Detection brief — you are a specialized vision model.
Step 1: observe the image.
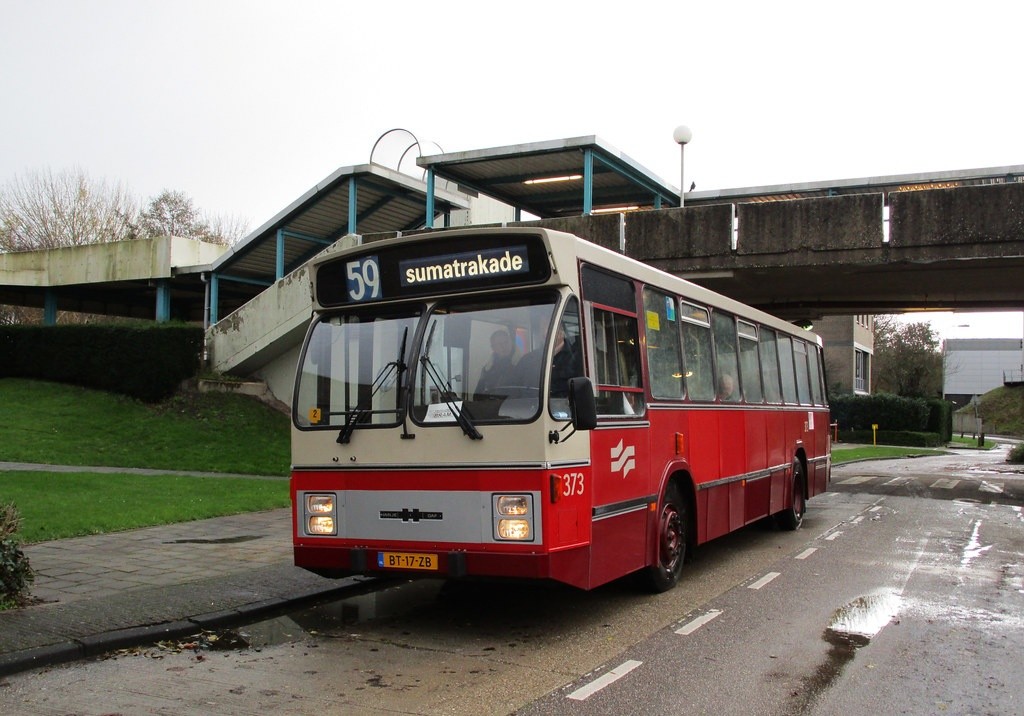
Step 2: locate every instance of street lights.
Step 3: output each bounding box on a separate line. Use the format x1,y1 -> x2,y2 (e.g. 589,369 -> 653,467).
673,125 -> 693,208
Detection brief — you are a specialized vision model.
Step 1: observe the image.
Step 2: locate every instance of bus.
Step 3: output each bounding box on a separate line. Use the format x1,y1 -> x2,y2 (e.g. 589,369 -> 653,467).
287,225 -> 833,596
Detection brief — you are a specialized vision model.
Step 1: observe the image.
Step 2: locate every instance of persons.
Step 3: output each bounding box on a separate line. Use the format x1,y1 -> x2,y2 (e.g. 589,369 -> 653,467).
473,317 -> 587,401
718,373 -> 738,400
